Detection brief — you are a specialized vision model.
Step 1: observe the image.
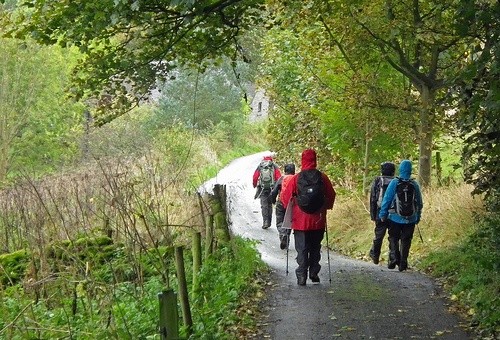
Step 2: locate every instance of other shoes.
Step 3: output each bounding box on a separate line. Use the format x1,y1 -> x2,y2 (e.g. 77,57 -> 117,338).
262,220 -> 268,229
309,273 -> 320,282
280,235 -> 287,249
399,264 -> 406,271
269,223 -> 271,226
369,249 -> 379,264
299,278 -> 305,286
388,258 -> 399,269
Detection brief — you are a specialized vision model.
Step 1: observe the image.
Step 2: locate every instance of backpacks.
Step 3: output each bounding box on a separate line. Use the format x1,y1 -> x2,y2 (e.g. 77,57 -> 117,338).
377,177 -> 395,212
259,162 -> 275,190
395,176 -> 416,217
293,170 -> 325,213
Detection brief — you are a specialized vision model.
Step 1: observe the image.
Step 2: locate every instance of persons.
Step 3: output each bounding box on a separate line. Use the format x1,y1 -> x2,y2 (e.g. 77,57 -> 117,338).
378,160 -> 422,272
280,149 -> 337,285
370,163 -> 400,266
270,163 -> 295,249
253,156 -> 281,229
